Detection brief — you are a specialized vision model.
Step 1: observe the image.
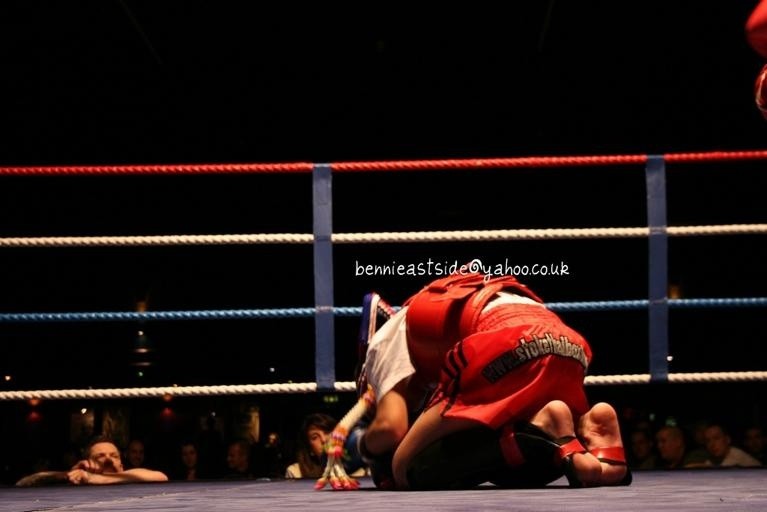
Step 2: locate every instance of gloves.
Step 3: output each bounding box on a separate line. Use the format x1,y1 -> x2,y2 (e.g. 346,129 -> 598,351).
346,427 -> 374,469
